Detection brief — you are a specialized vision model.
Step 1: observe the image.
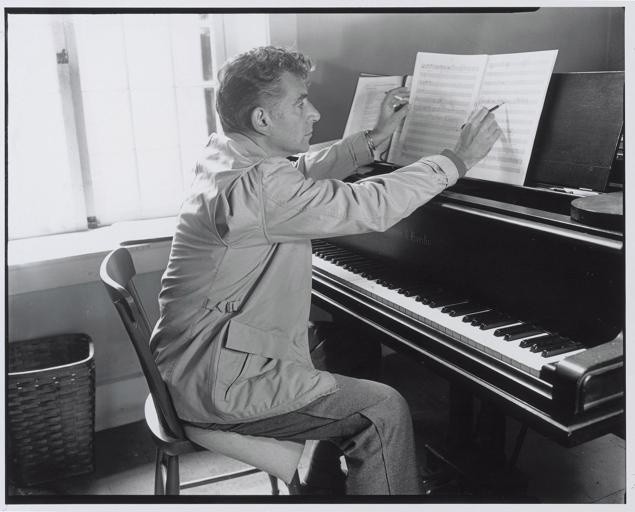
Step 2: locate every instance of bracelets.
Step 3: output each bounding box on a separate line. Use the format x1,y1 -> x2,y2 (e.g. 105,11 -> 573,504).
364,130 -> 376,150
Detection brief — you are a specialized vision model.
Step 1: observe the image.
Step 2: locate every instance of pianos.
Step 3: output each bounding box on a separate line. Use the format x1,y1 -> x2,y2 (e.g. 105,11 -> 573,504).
311,71 -> 626,503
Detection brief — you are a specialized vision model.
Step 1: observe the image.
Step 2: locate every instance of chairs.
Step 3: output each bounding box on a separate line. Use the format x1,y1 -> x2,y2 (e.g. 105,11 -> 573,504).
98,244 -> 318,498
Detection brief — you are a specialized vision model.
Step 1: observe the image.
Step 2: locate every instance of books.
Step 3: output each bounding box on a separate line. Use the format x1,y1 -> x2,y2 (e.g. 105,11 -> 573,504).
393,48 -> 560,186
342,72 -> 413,165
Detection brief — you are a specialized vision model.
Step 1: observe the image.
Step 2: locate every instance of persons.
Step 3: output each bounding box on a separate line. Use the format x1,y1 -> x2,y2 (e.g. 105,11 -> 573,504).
147,45 -> 503,498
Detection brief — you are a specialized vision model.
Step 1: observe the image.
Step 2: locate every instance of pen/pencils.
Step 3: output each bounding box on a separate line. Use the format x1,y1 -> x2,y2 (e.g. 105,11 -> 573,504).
460,102 -> 508,129
